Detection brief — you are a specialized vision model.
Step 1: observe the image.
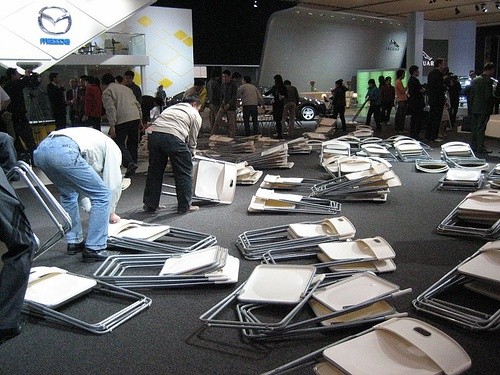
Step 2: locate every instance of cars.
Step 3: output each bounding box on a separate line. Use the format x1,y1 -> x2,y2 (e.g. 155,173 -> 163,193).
456,76 -> 471,107
163,91 -> 185,110
236,85 -> 326,121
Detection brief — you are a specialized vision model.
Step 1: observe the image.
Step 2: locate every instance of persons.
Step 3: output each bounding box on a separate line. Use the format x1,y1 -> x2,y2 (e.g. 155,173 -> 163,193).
394,70 -> 409,134
324,79 -> 348,134
143,94 -> 203,214
282,80 -> 299,138
307,81 -> 316,92
407,65 -> 427,141
365,76 -> 395,137
33,126 -> 123,263
207,70 -> 242,138
264,75 -> 288,138
464,62 -> 500,153
0,131 -> 36,344
141,95 -> 164,136
427,58 -> 462,142
171,85 -> 201,105
157,85 -> 167,112
1,68 -> 142,177
236,76 -> 263,136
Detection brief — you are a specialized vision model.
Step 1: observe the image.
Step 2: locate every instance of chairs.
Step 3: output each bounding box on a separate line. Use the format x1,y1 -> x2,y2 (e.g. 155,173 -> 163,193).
0,111 -> 500,375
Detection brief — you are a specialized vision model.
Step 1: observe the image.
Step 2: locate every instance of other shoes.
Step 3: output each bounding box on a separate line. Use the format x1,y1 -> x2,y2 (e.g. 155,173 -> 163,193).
143,203 -> 161,211
124,162 -> 139,178
178,205 -> 200,214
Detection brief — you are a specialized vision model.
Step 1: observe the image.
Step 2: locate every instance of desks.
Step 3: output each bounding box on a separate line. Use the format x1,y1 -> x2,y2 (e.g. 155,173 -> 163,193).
300,90 -> 332,102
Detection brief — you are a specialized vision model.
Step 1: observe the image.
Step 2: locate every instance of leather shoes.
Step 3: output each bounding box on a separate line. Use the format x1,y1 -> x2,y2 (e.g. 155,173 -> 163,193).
0,324 -> 23,345
82,249 -> 121,263
66,240 -> 86,255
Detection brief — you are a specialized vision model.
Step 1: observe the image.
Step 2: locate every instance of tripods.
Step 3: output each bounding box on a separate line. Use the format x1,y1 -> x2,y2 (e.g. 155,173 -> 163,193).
26,86 -> 50,145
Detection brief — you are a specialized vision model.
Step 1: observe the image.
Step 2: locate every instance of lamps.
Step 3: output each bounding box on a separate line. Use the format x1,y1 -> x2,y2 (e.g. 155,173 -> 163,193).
475,4 -> 480,10
454,7 -> 461,15
481,3 -> 489,12
495,1 -> 500,10
